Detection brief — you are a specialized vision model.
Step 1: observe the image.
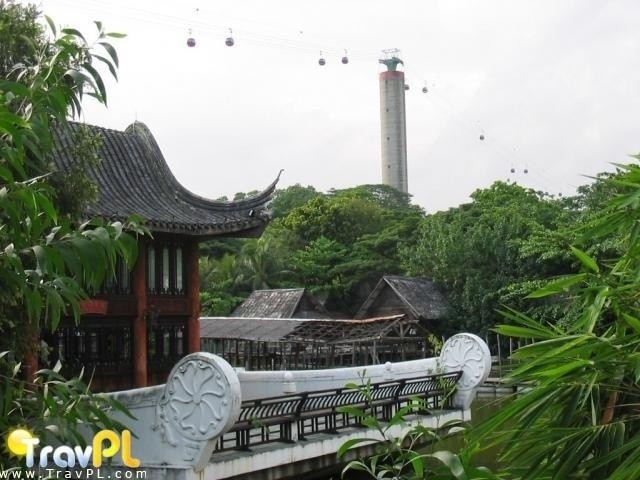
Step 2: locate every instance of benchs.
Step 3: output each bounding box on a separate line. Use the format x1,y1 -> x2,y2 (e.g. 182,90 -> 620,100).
213,370 -> 466,456
